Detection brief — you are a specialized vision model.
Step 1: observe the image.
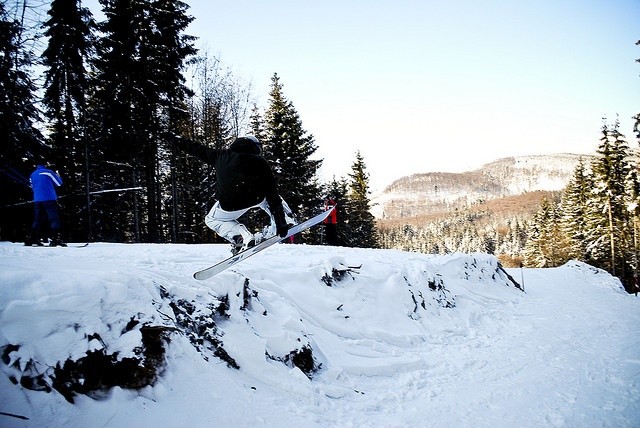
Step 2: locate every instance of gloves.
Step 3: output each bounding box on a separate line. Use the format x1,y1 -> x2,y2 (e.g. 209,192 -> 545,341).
159,130 -> 183,148
276,220 -> 291,237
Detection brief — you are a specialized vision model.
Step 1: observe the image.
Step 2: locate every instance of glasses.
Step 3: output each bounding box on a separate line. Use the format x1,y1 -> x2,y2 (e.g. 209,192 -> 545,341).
254,139 -> 264,144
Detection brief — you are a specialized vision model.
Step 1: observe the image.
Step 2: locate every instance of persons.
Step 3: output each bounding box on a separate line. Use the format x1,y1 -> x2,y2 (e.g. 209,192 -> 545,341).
25,158 -> 64,246
159,131 -> 298,256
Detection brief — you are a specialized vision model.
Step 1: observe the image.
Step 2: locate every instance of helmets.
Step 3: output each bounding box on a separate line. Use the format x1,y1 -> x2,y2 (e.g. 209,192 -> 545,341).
239,135 -> 260,142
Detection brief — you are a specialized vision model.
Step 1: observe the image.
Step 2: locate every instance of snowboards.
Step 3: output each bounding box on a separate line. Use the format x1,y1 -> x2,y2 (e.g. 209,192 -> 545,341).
23,242 -> 89,247
194,202 -> 337,281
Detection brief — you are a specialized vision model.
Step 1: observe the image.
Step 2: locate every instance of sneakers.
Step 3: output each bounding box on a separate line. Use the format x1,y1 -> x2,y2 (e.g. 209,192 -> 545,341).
230,234 -> 255,256
28,238 -> 44,245
49,239 -> 66,246
261,213 -> 295,241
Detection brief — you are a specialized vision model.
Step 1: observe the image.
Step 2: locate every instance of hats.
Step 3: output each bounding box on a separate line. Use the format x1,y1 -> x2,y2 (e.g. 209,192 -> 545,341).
35,158 -> 47,166
